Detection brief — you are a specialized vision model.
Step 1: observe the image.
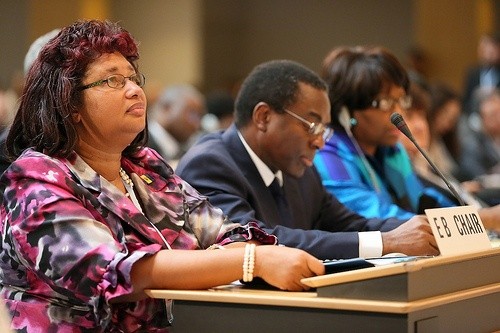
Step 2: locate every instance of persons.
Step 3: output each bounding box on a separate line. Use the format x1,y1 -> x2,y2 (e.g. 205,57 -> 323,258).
177,59 -> 440,262
0,18 -> 325,333
313,46 -> 500,235
0,29 -> 500,210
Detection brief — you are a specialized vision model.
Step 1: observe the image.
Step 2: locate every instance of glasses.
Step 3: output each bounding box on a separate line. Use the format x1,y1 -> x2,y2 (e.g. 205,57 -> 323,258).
76,73 -> 146,92
367,95 -> 412,112
282,108 -> 334,143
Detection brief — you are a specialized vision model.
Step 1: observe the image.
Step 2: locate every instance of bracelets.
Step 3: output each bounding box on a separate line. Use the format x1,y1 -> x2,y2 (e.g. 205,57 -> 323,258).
205,243 -> 224,250
243,243 -> 256,282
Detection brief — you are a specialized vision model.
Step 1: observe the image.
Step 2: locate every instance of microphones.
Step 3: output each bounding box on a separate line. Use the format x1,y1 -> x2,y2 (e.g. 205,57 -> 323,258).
389,112 -> 465,206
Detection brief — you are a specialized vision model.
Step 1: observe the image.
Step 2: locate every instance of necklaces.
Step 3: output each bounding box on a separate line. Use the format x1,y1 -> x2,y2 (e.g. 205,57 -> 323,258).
119,167 -> 134,199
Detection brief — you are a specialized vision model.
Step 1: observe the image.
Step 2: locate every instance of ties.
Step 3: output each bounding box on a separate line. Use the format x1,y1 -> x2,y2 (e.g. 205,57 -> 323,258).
268,176 -> 290,226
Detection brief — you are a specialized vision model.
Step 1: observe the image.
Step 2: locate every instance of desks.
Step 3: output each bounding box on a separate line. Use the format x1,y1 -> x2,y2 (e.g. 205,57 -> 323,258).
151,248 -> 500,333
